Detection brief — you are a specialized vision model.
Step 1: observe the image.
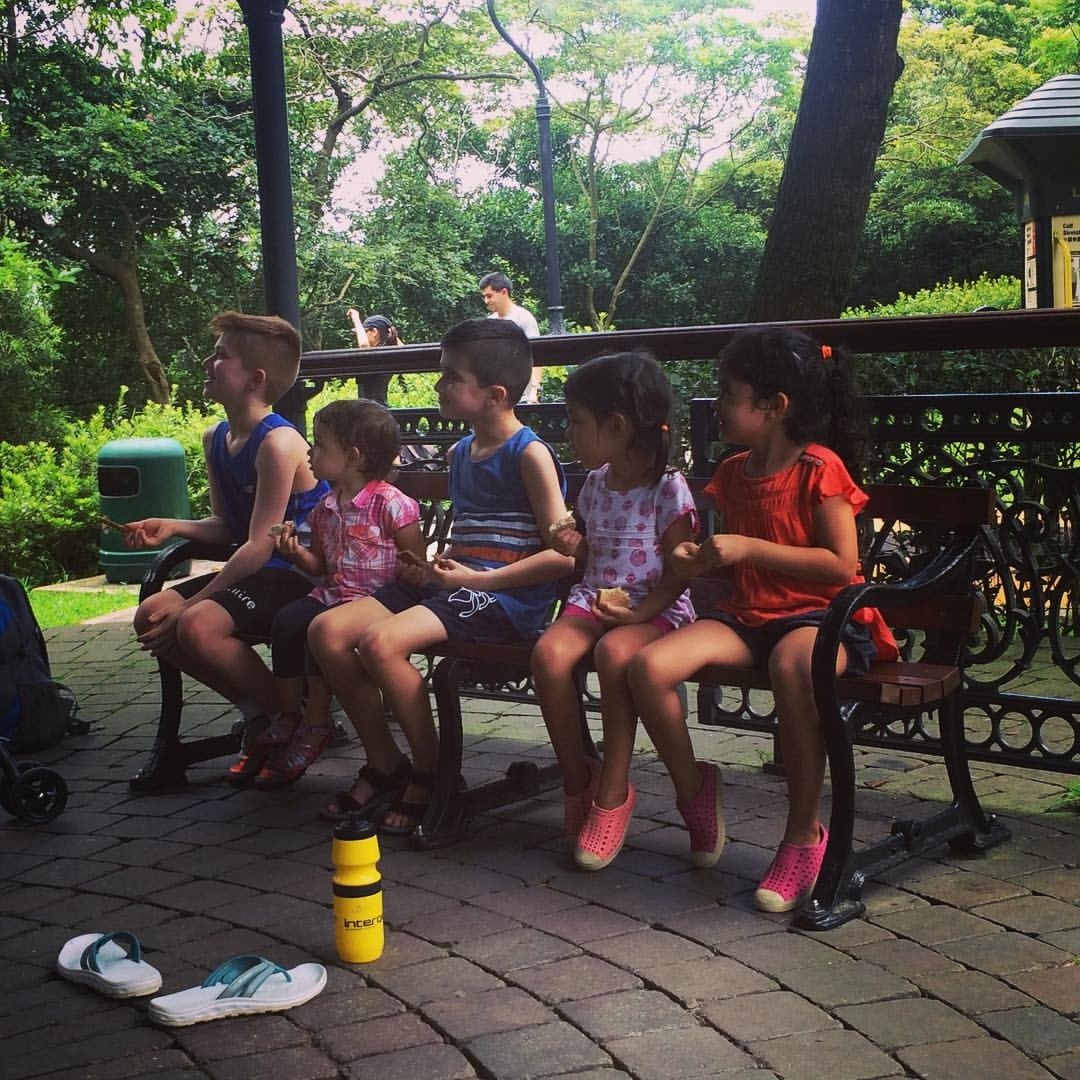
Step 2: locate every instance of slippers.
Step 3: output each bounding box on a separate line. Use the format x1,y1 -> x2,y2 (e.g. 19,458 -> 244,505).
57,931 -> 162,1001
149,954 -> 328,1028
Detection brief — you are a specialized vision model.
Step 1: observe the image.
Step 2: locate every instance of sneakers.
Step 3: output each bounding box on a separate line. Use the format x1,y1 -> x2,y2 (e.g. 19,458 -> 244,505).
228,714 -> 272,781
253,712 -> 304,793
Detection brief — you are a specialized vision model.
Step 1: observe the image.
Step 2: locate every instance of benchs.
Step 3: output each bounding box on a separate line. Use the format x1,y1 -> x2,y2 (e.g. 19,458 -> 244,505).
129,472 -> 996,933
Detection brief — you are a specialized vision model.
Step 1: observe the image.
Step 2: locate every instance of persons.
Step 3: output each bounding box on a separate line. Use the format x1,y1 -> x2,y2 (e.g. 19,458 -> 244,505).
479,271 -> 543,405
346,307 -> 407,408
627,327 -> 899,913
531,351 -> 695,873
308,319 -> 577,833
122,310 -> 331,789
256,400 -> 427,772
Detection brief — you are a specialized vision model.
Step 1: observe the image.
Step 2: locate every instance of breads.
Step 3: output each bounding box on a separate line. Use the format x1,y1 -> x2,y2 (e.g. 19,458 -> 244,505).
268,520 -> 297,539
548,516 -> 577,535
597,586 -> 632,610
396,549 -> 421,565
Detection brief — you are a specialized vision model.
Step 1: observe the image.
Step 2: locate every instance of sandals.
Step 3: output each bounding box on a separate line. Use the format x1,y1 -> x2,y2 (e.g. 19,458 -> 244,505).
318,753 -> 412,818
379,771 -> 435,833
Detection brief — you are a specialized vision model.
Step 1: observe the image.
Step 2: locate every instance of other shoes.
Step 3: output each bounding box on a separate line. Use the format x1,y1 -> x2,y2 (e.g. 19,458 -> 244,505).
267,707 -> 308,746
564,760 -> 603,842
572,783 -> 635,870
283,718 -> 336,770
753,823 -> 829,912
676,762 -> 725,868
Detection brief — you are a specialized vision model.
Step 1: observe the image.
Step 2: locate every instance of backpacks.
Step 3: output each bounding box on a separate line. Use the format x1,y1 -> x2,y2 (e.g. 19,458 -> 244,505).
0,574 -> 89,754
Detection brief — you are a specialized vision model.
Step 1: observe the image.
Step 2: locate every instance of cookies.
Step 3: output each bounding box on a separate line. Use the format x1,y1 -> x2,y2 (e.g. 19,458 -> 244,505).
97,516 -> 125,529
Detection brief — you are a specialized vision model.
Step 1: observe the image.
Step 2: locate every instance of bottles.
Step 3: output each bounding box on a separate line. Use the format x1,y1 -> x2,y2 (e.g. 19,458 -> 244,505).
330,808 -> 385,965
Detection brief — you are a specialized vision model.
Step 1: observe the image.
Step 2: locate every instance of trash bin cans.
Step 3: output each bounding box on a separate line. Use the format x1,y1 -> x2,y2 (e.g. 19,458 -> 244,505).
94,437 -> 193,581
